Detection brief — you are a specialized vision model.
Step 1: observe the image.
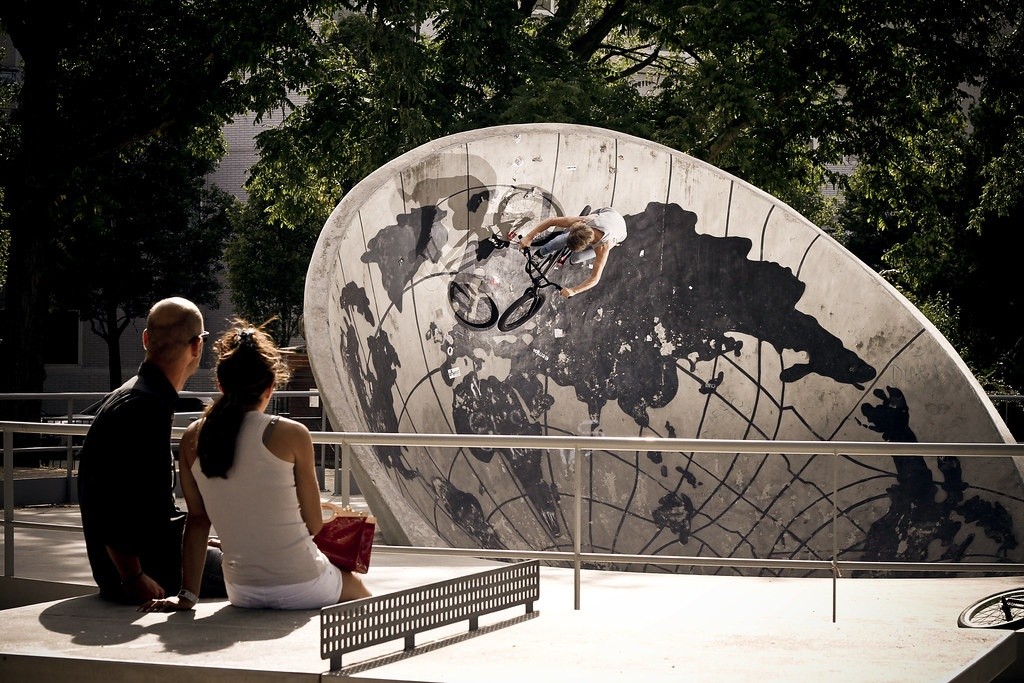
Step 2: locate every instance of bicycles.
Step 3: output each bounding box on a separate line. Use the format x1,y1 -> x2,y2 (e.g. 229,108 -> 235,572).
498,205 -> 591,332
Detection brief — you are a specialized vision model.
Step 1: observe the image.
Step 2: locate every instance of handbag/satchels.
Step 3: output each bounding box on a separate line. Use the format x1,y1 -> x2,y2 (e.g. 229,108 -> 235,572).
308,502 -> 377,574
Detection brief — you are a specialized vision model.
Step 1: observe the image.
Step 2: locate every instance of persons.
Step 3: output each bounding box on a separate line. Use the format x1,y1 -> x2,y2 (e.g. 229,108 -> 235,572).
516,206 -> 629,298
77,296 -> 373,613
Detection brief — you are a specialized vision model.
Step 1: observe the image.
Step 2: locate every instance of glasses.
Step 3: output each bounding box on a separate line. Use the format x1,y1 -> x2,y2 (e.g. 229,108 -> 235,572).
188,331 -> 210,345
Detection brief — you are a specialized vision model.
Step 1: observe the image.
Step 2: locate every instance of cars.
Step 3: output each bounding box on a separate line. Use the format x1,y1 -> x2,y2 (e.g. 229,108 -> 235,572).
48,390 -> 215,450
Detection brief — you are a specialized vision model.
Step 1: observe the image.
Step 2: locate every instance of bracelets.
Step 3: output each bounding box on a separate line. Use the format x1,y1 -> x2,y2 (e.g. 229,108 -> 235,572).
120,572 -> 143,583
177,588 -> 199,604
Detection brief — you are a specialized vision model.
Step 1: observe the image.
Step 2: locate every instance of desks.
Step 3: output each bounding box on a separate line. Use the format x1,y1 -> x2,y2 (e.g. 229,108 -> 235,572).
0,551 -> 1024,683
